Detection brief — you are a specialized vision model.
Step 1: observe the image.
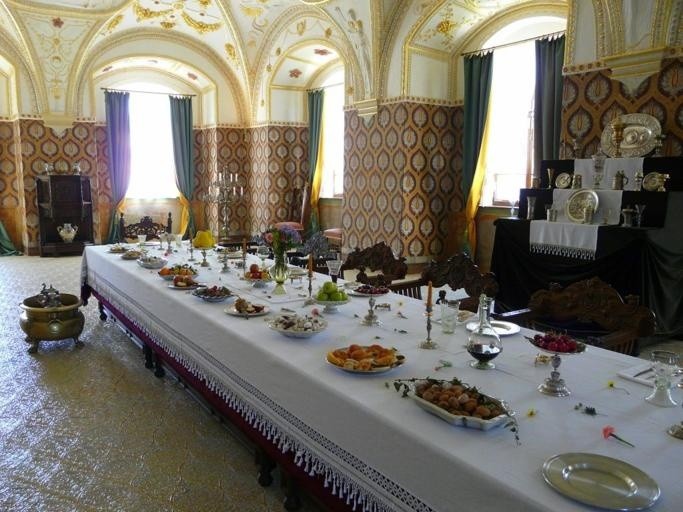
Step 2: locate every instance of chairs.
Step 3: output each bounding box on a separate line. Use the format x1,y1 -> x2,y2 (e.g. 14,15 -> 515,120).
119,211 -> 172,242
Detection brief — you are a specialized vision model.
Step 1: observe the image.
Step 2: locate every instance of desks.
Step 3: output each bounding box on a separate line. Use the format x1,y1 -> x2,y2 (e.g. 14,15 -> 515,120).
78,238 -> 683,512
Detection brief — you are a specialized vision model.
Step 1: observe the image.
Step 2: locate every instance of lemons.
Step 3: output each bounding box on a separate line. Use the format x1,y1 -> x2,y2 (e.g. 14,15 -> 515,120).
193,230 -> 214,247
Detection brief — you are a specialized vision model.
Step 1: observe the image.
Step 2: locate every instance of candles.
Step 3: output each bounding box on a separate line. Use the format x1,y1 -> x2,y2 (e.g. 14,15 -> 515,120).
208,172 -> 244,197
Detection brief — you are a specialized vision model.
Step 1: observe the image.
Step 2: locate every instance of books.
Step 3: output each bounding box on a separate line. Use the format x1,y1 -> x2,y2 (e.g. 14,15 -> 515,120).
616,361 -> 682,389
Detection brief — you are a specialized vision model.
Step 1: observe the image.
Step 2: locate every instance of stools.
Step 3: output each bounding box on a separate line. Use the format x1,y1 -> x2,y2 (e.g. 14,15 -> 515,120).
274,221 -> 305,240
324,227 -> 342,252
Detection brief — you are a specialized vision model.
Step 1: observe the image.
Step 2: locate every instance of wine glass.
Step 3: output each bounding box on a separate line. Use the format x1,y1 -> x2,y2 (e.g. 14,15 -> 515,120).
525,169 -> 670,229
590,154 -> 606,189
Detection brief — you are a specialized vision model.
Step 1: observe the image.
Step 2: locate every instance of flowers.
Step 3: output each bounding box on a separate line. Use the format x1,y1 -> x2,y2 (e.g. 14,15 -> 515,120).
259,222 -> 303,277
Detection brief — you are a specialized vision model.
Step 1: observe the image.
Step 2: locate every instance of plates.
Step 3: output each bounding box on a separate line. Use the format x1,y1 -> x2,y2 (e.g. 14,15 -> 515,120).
410,379 -> 514,433
465,320 -> 523,336
312,294 -> 352,314
541,452 -> 661,510
326,345 -> 407,376
600,113 -> 662,158
530,340 -> 589,396
350,286 -> 390,327
269,314 -> 328,340
223,303 -> 271,317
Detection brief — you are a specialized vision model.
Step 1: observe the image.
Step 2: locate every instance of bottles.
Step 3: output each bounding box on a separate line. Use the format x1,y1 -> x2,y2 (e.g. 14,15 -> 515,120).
467,296 -> 505,370
72,162 -> 82,176
44,164 -> 56,176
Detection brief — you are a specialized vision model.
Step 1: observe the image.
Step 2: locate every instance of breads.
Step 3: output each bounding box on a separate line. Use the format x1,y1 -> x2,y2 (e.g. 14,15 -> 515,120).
160,268 -> 169,274
328,344 -> 394,371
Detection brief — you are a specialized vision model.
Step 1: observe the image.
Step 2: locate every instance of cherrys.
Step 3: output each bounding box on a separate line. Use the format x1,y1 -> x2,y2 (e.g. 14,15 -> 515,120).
356,281 -> 387,294
534,329 -> 581,352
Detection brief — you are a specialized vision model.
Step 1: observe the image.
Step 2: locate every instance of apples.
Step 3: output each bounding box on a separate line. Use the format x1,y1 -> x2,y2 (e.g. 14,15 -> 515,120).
316,283 -> 348,302
245,264 -> 269,279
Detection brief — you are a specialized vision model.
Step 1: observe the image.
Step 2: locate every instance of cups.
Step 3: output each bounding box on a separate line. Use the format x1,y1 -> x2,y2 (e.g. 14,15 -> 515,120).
645,349 -> 681,408
439,300 -> 462,334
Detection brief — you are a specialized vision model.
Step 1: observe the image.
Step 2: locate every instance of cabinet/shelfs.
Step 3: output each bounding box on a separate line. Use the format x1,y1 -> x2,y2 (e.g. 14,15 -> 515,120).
35,173 -> 94,257
491,156 -> 683,357
